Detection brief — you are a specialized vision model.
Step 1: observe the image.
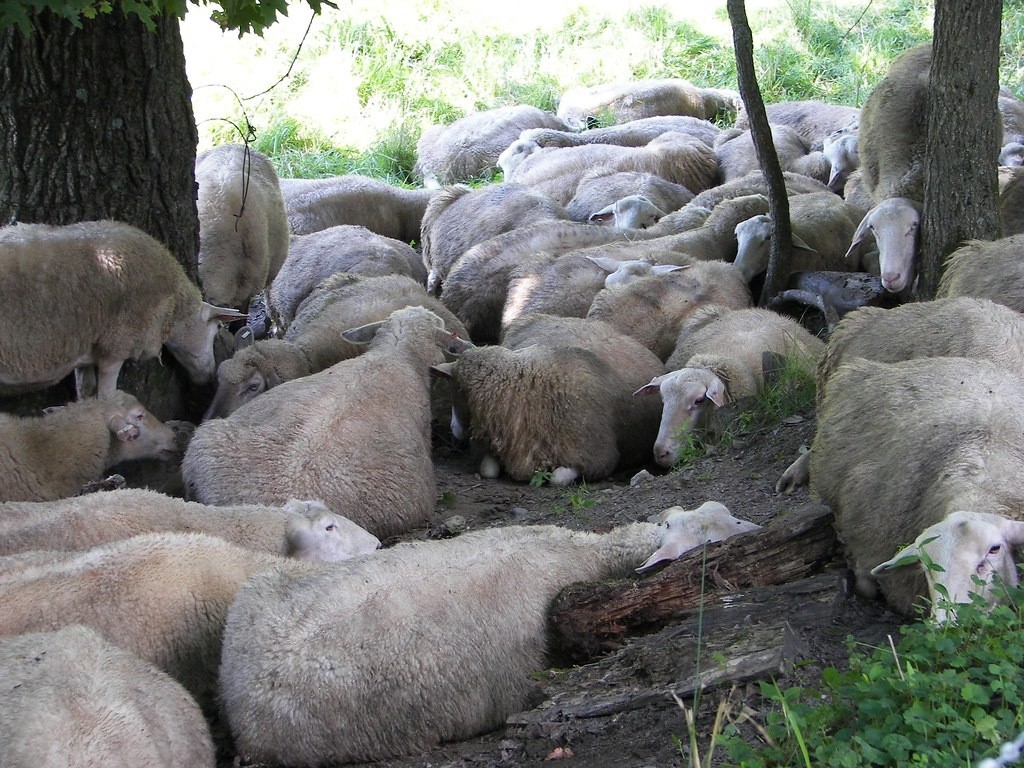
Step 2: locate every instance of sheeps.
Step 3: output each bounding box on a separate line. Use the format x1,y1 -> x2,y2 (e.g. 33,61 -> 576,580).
0,42 -> 1024,768
